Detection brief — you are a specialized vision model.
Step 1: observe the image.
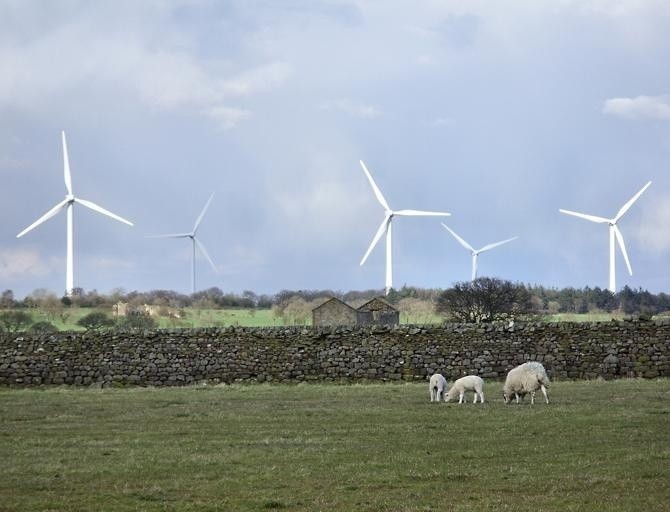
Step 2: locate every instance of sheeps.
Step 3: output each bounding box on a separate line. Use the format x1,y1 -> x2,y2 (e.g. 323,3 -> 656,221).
444,375 -> 485,404
429,373 -> 449,404
502,362 -> 553,407
